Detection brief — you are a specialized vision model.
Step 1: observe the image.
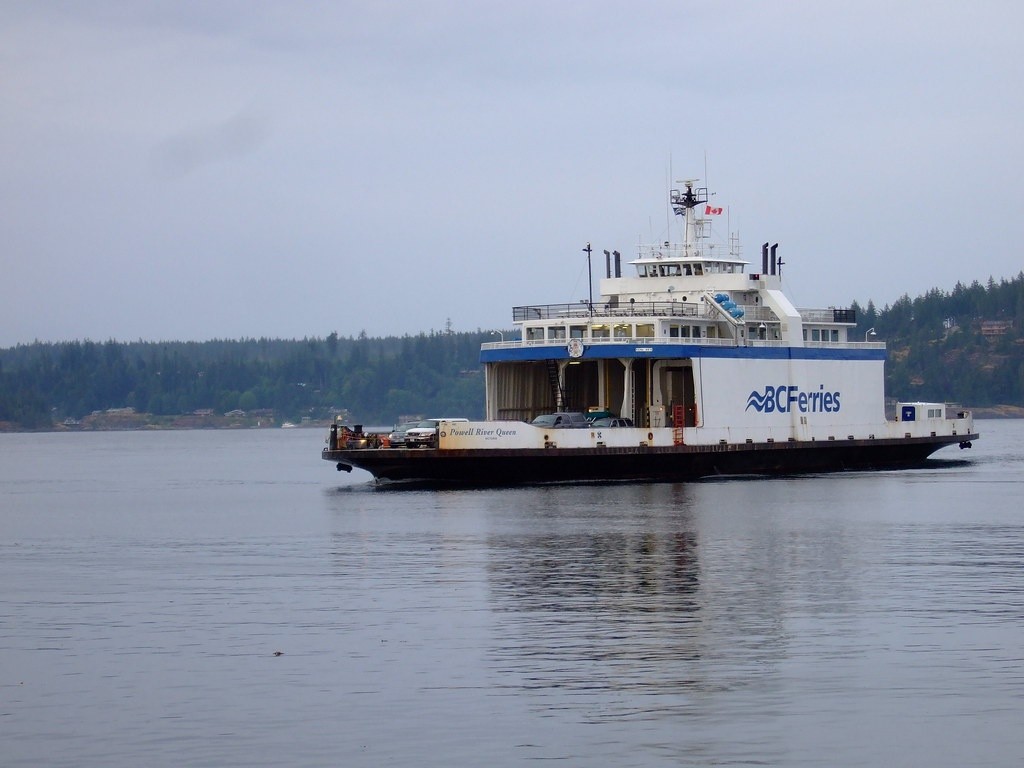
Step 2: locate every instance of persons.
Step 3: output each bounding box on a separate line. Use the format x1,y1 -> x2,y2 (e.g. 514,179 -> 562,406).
675,265 -> 681,273
660,266 -> 664,275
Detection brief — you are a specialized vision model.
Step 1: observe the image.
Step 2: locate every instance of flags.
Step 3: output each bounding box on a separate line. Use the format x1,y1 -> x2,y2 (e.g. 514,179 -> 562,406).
705,205 -> 722,215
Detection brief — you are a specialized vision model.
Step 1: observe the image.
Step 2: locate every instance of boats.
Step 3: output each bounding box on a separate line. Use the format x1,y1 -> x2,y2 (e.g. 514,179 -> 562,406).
322,149 -> 980,484
